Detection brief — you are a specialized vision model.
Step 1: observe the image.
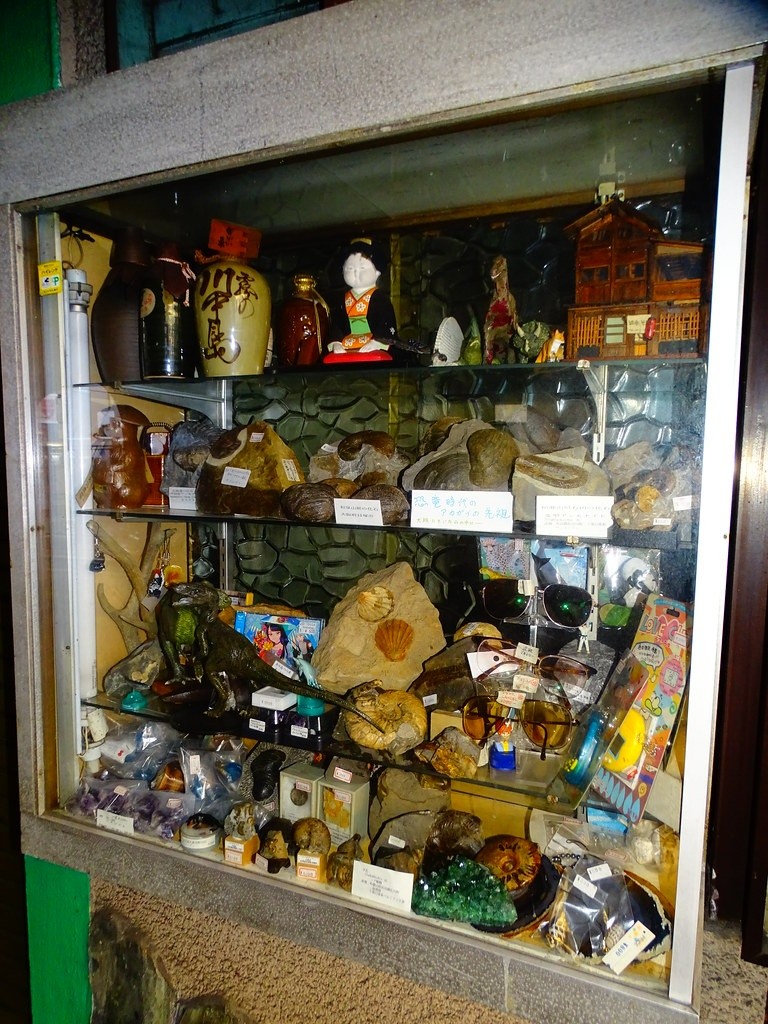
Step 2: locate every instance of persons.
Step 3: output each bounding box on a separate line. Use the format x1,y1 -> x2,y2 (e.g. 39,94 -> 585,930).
322,238 -> 396,363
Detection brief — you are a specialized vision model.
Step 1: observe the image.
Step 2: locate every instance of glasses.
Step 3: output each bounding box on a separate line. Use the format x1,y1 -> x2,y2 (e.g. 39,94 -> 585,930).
481,578 -> 592,628
476,637 -> 598,700
462,695 -> 583,760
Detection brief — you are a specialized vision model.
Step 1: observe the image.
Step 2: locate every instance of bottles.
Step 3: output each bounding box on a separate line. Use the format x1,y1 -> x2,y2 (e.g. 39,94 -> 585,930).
90,225 -> 330,384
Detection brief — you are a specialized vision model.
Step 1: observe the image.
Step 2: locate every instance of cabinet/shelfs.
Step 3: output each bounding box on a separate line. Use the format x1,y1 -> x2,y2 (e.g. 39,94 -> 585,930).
50,61 -> 728,989
